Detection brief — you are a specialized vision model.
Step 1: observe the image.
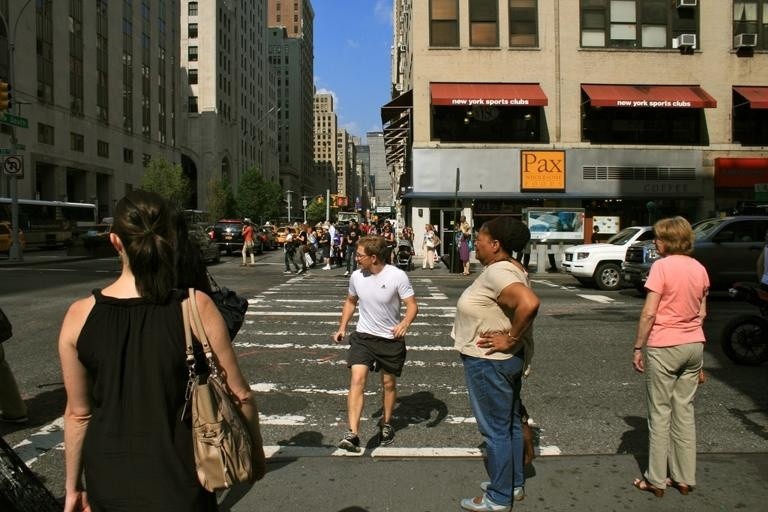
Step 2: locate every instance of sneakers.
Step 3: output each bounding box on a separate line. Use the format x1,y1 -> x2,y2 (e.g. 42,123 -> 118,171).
461,493 -> 512,511
378,423 -> 393,446
480,482 -> 524,500
283,265 -> 350,276
338,432 -> 362,452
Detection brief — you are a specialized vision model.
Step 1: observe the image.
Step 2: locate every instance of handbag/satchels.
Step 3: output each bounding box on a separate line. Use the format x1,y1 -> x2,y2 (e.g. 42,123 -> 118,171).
246,240 -> 254,249
207,286 -> 248,340
188,355 -> 254,493
522,421 -> 535,466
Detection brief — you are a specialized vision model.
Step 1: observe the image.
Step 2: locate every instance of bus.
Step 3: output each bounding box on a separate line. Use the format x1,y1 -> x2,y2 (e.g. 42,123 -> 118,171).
0,198 -> 98,252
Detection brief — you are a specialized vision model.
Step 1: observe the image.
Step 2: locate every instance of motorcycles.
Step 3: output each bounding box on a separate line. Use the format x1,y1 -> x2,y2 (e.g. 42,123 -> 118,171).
720,280 -> 767,365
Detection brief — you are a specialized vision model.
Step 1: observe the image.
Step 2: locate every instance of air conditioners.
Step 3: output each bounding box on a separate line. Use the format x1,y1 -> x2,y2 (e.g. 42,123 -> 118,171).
676,0 -> 697,9
678,34 -> 695,49
395,0 -> 409,92
733,33 -> 758,49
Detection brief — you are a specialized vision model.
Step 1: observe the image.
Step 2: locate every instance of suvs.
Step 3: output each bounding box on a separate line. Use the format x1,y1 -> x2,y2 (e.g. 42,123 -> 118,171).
621,216 -> 767,291
561,224 -> 656,290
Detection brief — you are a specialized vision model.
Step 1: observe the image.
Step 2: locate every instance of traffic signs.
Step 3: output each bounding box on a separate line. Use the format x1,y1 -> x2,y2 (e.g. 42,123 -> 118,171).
0,114 -> 27,129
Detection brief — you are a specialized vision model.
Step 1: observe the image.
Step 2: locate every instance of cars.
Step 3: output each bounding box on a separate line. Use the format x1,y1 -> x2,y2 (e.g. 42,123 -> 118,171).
0,221 -> 27,257
182,216 -> 309,265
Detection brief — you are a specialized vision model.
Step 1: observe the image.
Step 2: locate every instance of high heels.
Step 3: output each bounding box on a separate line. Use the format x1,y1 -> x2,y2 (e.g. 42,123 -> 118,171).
666,477 -> 689,495
633,477 -> 664,497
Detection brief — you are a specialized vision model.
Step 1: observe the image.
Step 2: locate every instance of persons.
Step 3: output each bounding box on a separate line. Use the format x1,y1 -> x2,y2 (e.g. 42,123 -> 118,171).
238,215 -> 533,276
590,225 -> 600,243
754,241 -> 768,293
554,212 -> 579,230
163,212 -> 212,428
333,235 -> 418,453
58,188 -> 265,511
0,308 -> 29,423
631,216 -> 711,497
450,216 -> 541,512
519,402 -> 537,470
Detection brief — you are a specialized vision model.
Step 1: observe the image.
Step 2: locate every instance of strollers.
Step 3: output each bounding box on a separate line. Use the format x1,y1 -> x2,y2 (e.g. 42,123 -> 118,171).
393,238 -> 414,270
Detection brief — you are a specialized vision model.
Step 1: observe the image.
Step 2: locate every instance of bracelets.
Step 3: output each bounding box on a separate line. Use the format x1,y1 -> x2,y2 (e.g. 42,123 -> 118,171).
507,330 -> 521,341
634,346 -> 642,352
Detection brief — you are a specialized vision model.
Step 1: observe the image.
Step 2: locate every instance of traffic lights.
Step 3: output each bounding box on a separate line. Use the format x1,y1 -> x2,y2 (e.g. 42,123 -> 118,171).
335,196 -> 348,207
0,83 -> 12,111
